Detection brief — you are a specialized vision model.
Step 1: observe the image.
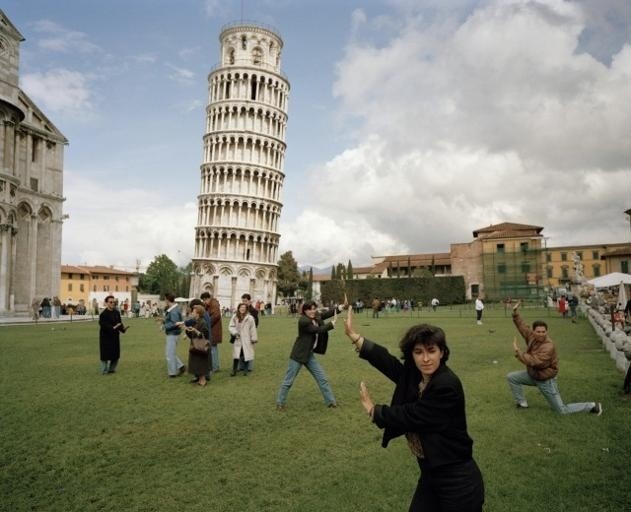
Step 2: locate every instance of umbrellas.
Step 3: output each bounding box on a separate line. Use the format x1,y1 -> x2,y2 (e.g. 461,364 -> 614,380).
586,272 -> 631,288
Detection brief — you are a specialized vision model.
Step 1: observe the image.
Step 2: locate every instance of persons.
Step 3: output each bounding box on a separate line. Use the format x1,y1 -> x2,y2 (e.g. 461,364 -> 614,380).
345,304 -> 485,511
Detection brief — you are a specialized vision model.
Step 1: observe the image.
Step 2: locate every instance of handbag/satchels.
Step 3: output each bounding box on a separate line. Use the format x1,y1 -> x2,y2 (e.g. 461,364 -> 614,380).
193,333 -> 208,351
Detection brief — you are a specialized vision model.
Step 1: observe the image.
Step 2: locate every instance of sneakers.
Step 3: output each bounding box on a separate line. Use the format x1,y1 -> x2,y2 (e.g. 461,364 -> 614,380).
591,402 -> 601,416
